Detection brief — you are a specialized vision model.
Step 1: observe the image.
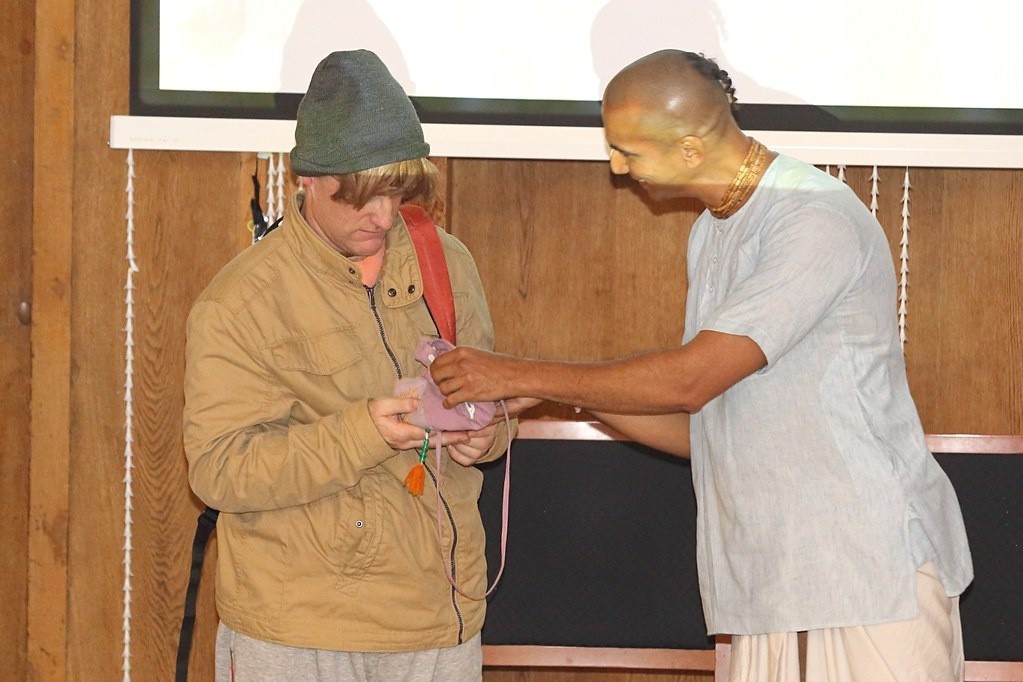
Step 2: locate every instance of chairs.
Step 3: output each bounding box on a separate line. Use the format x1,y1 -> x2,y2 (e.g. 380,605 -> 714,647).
472,415 -> 1023,682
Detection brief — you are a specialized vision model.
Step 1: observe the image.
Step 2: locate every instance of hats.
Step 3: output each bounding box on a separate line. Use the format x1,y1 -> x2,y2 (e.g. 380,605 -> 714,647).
289,48 -> 431,178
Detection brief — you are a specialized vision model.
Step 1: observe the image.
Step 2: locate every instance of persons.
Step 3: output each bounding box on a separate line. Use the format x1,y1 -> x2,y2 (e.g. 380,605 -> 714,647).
177,50 -> 518,682
431,48 -> 978,682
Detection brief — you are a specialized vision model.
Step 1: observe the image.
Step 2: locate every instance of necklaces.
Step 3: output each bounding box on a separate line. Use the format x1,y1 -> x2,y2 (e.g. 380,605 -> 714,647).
703,134 -> 769,219
